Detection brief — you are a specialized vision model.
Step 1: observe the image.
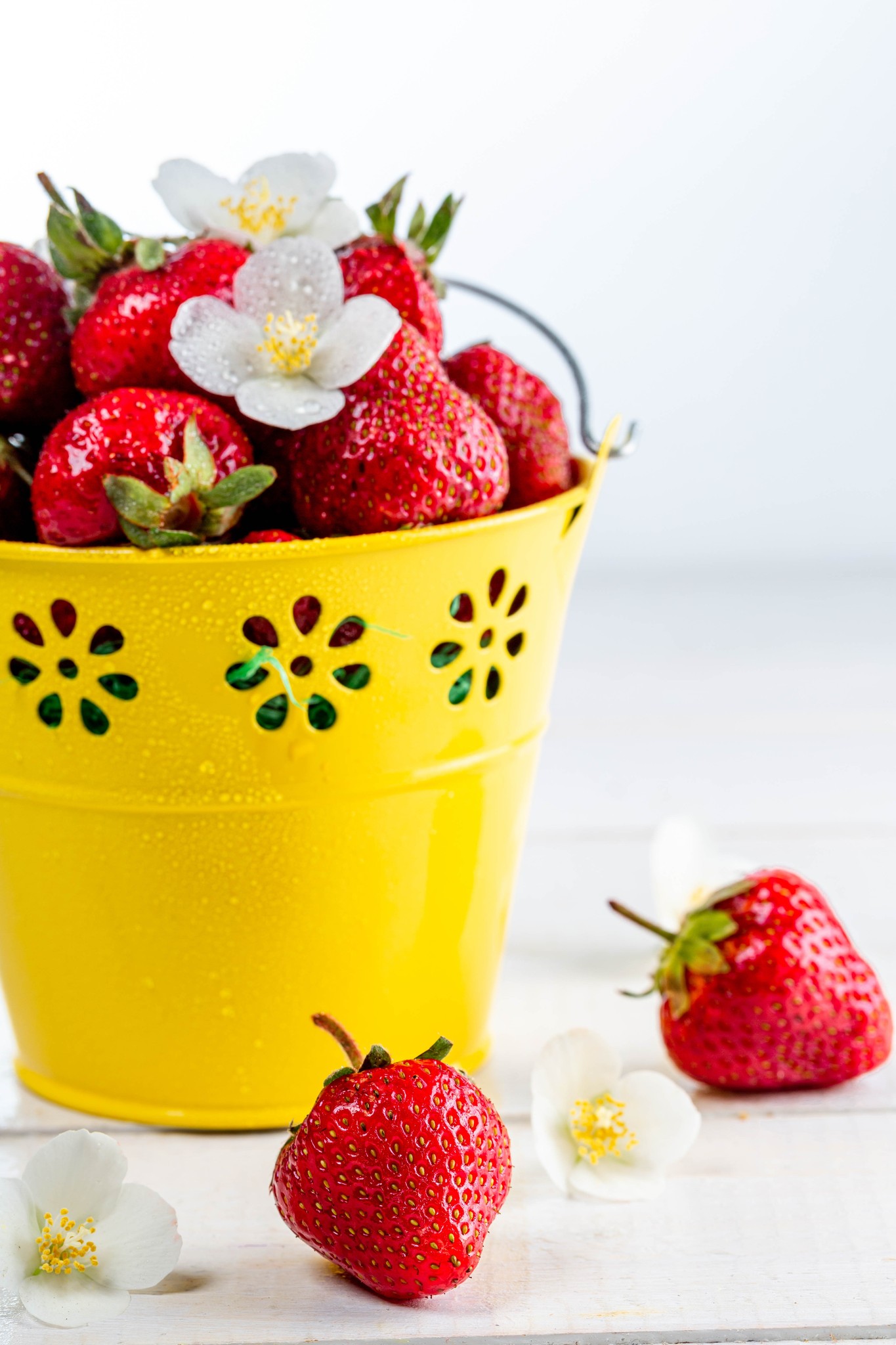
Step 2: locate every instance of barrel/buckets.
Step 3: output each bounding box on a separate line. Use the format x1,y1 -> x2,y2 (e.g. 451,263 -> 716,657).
1,270 -> 638,1131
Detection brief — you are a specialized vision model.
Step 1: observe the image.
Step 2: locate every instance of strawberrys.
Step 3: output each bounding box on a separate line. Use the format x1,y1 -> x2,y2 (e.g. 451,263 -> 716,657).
605,870 -> 896,1094
0,173 -> 577,672
266,1016 -> 512,1299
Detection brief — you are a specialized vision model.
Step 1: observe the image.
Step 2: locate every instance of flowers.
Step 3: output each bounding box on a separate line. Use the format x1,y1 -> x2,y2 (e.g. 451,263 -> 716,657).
530,1029 -> 702,1197
154,147 -> 399,426
0,1129 -> 180,1327
